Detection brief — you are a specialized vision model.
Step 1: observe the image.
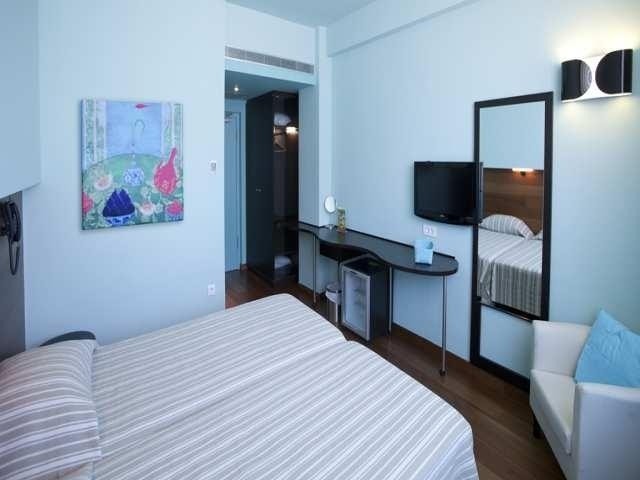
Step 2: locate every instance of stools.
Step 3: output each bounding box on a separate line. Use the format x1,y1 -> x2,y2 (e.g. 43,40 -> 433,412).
528,319 -> 638,480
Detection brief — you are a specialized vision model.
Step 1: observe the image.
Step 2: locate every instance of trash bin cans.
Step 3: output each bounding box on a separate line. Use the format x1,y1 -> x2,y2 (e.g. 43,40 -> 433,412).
325,281 -> 342,324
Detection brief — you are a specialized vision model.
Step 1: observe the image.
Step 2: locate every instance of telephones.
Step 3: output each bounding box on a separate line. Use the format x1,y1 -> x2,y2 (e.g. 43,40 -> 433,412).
0,201 -> 21,240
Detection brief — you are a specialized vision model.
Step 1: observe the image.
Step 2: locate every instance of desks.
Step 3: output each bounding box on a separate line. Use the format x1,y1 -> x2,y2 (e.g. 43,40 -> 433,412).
312,224 -> 458,376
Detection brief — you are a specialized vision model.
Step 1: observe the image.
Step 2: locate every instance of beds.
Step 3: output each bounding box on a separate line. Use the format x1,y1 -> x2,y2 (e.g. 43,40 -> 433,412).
0,293 -> 472,480
478,167 -> 544,315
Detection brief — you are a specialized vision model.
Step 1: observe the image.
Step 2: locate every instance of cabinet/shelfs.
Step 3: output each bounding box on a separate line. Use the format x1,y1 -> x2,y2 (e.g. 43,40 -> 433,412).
244,91 -> 299,291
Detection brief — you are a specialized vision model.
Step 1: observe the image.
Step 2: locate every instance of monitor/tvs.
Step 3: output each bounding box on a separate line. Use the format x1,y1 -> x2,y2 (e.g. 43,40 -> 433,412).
413,161 -> 484,225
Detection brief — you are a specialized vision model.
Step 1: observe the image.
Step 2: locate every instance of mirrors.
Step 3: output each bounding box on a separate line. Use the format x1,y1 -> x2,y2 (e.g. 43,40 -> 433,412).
468,91 -> 554,391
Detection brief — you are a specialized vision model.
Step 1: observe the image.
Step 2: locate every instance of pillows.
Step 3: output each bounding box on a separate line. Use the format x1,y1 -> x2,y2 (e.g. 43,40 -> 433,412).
479,213 -> 533,241
0,335 -> 103,480
574,306 -> 638,389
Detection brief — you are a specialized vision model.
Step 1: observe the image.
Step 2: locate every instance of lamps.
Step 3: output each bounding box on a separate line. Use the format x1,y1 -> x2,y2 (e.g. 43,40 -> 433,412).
559,46 -> 634,105
